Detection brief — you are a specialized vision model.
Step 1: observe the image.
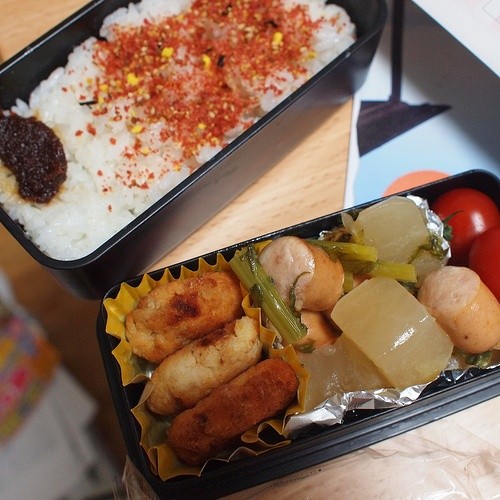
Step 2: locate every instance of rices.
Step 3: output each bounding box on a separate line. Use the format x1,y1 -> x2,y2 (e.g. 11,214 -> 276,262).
0,1 -> 353,261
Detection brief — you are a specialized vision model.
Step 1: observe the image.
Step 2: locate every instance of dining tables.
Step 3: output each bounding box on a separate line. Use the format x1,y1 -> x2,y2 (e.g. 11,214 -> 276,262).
1,1 -> 500,500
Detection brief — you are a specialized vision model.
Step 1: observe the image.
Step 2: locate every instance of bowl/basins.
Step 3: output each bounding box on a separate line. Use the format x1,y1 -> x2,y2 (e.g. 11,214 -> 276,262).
0,0 -> 401,303
95,166 -> 499,499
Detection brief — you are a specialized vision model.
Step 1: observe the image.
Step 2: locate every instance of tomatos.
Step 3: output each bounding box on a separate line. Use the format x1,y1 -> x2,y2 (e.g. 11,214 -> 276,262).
436,187 -> 500,301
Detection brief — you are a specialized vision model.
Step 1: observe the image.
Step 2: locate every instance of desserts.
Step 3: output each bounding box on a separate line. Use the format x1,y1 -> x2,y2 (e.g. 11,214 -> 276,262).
126,270 -> 299,465
